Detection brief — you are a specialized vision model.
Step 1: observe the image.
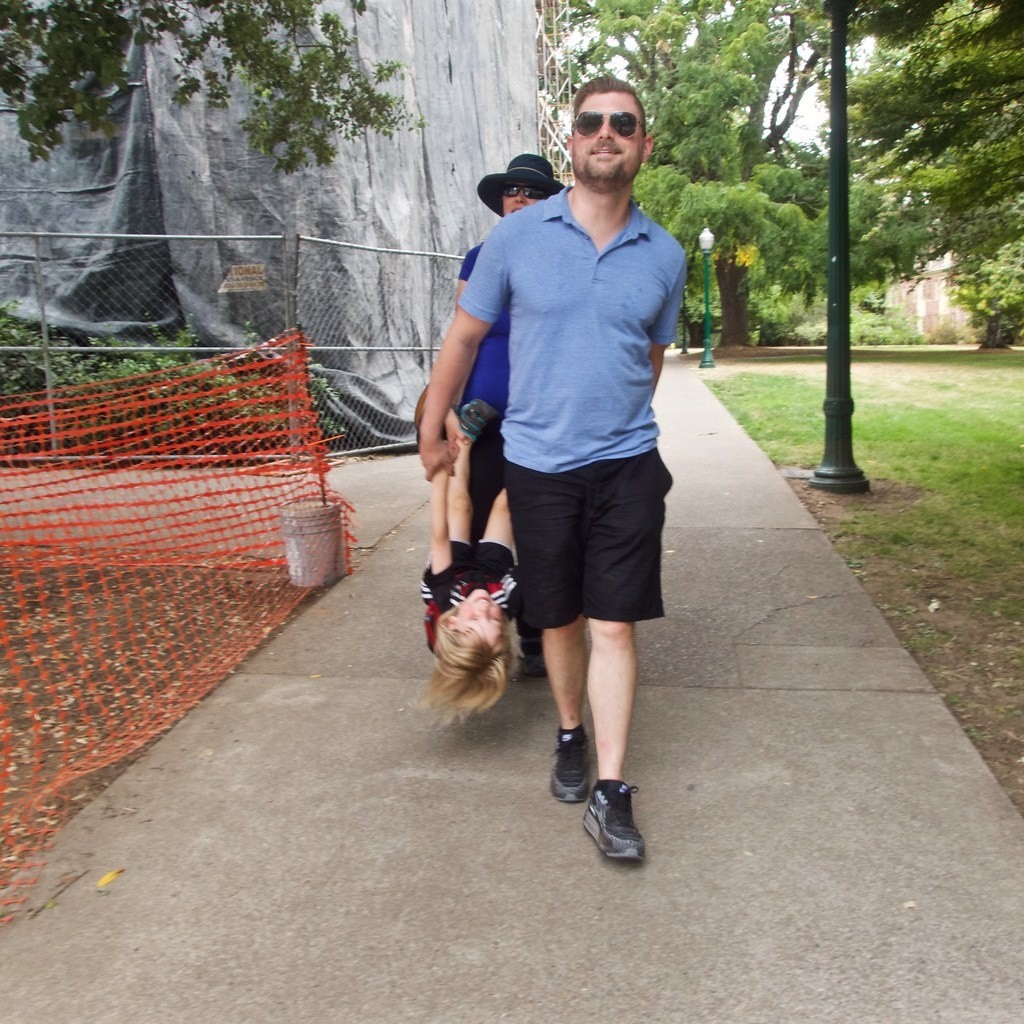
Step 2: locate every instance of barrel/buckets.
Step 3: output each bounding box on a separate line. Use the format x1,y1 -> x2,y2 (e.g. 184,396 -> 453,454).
279,496 -> 347,588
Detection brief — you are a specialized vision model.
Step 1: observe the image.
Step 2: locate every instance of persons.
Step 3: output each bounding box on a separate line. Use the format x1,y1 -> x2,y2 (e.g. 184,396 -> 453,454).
413,153 -> 568,726
420,76 -> 687,861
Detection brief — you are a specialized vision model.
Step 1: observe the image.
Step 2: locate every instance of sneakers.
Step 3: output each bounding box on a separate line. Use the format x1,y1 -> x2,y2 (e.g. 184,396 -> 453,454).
581,782 -> 645,859
549,729 -> 591,803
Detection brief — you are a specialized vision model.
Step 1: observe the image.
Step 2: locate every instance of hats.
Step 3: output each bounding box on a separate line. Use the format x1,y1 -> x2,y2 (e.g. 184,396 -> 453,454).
477,153 -> 566,220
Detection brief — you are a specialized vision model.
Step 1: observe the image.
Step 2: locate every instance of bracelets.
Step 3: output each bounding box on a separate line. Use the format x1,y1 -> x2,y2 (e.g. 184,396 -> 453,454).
451,403 -> 461,416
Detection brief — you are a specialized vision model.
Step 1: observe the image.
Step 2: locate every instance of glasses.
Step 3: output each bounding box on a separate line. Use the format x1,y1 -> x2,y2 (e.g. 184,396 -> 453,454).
499,184 -> 548,199
571,110 -> 646,140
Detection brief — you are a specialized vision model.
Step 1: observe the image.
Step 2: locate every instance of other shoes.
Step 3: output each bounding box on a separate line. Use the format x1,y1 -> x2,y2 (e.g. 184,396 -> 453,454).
519,635 -> 548,678
460,397 -> 500,441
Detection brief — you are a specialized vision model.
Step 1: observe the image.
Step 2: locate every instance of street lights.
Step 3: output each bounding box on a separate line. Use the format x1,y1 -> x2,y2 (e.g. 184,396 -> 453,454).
701,227 -> 716,368
